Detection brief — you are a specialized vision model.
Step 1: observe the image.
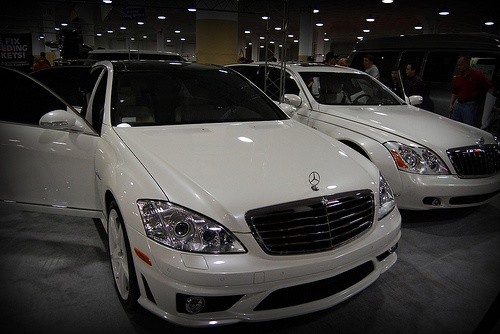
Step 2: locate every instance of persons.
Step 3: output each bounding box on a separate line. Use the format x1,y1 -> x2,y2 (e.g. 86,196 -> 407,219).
307,56 -> 313,62
239,57 -> 251,64
273,58 -> 277,62
307,51 -> 379,104
448,54 -> 494,126
35,52 -> 51,71
390,62 -> 434,112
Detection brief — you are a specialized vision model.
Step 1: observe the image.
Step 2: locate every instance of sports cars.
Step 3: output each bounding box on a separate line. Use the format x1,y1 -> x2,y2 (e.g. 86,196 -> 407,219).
1,43 -> 402,334
221,59 -> 500,215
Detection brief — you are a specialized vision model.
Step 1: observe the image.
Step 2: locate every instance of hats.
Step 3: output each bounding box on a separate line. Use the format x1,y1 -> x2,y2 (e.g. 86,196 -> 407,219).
326,51 -> 339,58
